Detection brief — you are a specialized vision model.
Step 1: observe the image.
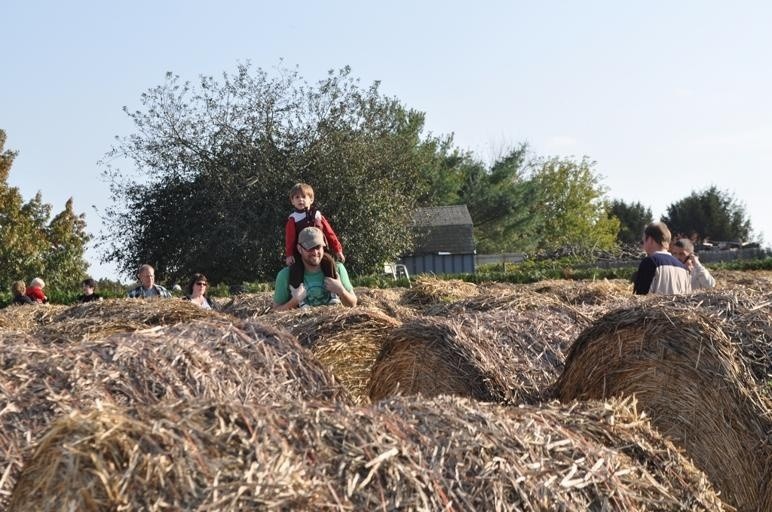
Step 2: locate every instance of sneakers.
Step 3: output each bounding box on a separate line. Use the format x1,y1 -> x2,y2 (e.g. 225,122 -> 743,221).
330,292 -> 341,303
299,301 -> 309,308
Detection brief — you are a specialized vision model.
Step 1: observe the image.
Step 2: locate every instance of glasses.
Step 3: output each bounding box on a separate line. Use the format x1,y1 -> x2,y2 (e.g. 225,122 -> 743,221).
194,281 -> 209,287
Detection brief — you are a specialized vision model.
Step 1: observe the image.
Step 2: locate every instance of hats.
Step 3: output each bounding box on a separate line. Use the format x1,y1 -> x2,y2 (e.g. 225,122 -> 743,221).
297,226 -> 327,250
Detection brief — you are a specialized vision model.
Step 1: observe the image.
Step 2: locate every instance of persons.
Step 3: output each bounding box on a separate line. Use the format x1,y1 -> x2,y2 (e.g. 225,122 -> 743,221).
76,277 -> 100,302
11,280 -> 34,305
179,271 -> 213,311
26,277 -> 49,304
634,221 -> 693,295
284,182 -> 345,308
272,225 -> 358,311
671,238 -> 717,291
123,263 -> 172,298
